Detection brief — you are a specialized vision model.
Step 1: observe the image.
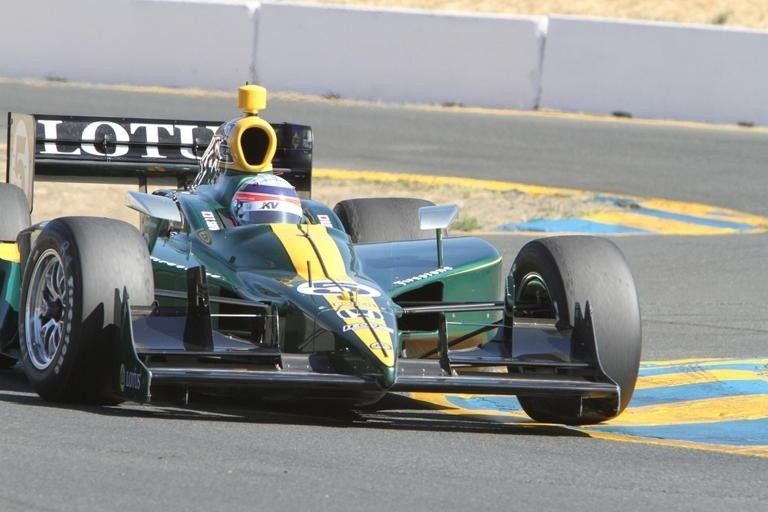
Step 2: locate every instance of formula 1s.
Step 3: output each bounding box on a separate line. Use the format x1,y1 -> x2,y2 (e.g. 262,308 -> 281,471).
2,83 -> 641,427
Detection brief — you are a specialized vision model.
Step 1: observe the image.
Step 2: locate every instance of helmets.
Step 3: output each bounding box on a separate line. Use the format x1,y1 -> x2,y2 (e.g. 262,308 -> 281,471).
230,174 -> 303,225
